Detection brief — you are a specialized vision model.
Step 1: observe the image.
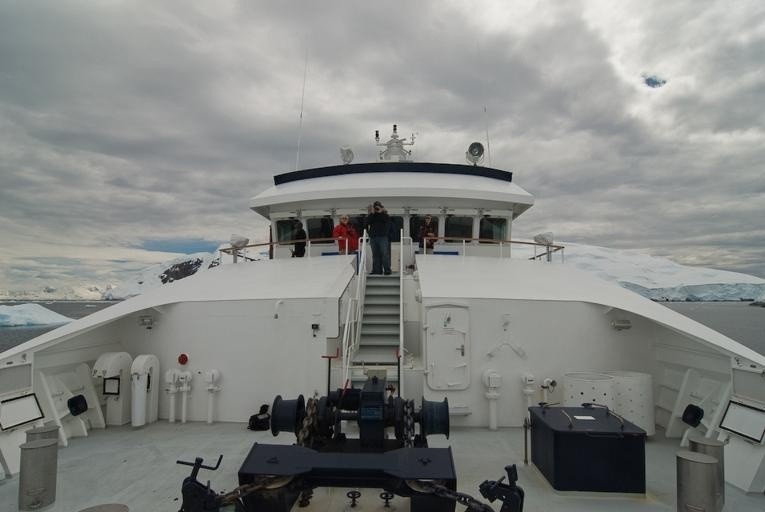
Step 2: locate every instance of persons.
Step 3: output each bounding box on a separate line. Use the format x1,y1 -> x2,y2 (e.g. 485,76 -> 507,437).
417,214 -> 438,254
365,201 -> 392,275
332,214 -> 358,255
292,222 -> 306,257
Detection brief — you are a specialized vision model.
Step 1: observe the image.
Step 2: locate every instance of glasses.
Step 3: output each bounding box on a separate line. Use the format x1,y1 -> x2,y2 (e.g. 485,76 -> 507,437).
425,217 -> 430,219
342,218 -> 347,220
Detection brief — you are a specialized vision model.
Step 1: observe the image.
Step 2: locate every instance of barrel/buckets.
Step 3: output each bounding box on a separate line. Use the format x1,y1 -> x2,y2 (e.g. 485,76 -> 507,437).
676,451 -> 722,512
18,438 -> 58,512
689,436 -> 724,503
25,425 -> 61,442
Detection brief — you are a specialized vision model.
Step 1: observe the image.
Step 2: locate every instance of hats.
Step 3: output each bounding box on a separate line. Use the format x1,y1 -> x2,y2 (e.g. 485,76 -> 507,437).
373,201 -> 384,209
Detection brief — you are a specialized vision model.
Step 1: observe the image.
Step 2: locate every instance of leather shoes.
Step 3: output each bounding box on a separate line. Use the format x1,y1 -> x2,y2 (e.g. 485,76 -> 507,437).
370,272 -> 392,275
